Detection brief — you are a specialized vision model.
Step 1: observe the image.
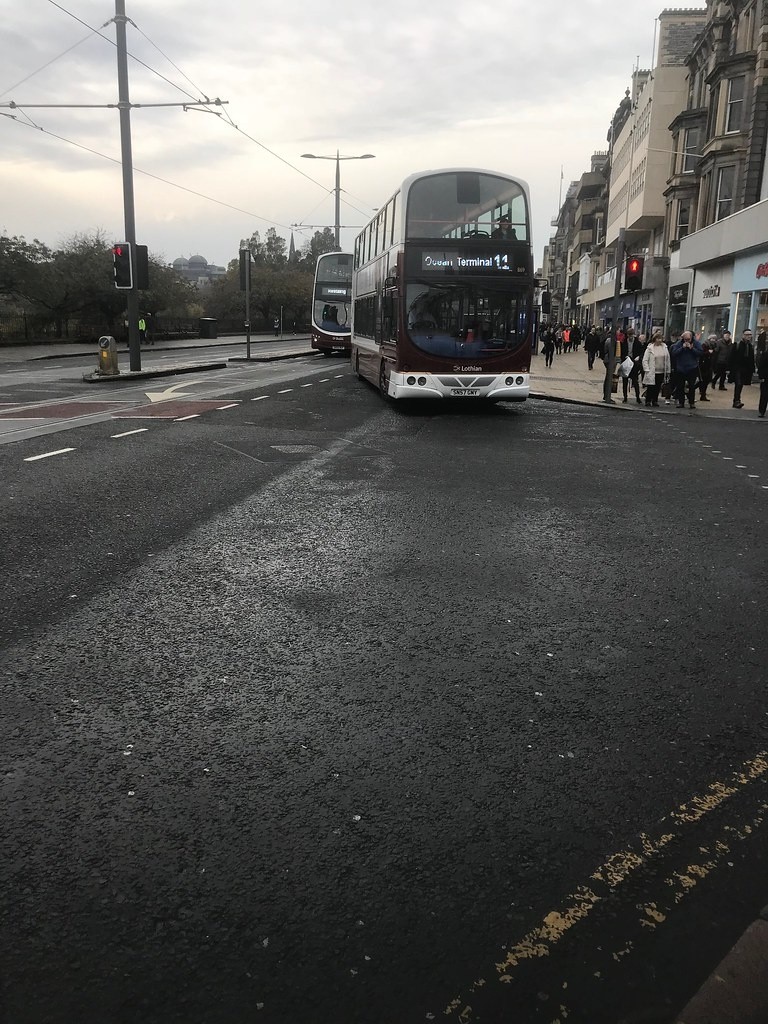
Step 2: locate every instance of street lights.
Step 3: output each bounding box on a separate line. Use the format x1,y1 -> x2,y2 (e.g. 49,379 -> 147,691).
300,153 -> 376,252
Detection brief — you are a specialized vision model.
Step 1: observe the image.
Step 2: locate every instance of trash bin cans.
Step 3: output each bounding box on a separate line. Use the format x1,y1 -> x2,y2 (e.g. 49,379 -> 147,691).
199,317 -> 218,339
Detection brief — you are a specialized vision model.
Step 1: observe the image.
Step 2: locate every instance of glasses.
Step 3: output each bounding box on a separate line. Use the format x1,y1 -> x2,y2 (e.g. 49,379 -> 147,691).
744,334 -> 752,336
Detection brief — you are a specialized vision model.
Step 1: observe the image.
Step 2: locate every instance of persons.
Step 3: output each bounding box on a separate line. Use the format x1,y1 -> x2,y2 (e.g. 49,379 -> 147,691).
491,219 -> 517,240
540,321 -> 768,418
408,300 -> 438,328
292,320 -> 297,335
273,317 -> 279,337
125,313 -> 154,347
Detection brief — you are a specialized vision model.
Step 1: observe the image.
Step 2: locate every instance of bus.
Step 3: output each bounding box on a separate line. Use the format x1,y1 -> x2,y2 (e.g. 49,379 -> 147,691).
311,252 -> 354,356
351,167 -> 552,402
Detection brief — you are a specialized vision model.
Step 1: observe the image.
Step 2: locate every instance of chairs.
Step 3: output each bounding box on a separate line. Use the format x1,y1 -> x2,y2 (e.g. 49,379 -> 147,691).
462,230 -> 493,239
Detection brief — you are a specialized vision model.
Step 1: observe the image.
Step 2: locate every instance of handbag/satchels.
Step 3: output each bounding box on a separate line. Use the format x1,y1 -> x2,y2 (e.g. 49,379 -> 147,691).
541,345 -> 546,354
611,374 -> 618,393
661,383 -> 669,397
619,357 -> 634,377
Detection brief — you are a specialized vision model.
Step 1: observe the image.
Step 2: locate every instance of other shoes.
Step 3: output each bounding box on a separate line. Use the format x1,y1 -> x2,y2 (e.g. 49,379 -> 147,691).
603,397 -> 615,404
700,396 -> 710,401
758,412 -> 765,418
636,398 -> 642,403
665,398 -> 670,405
652,402 -> 659,407
733,400 -> 744,409
645,402 -> 651,406
712,379 -> 716,389
719,385 -> 727,390
690,404 -> 696,409
676,404 -> 684,408
622,399 -> 627,403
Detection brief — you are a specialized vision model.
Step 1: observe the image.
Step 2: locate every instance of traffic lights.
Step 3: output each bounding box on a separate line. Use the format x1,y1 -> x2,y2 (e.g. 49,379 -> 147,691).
625,257 -> 644,289
113,242 -> 133,290
